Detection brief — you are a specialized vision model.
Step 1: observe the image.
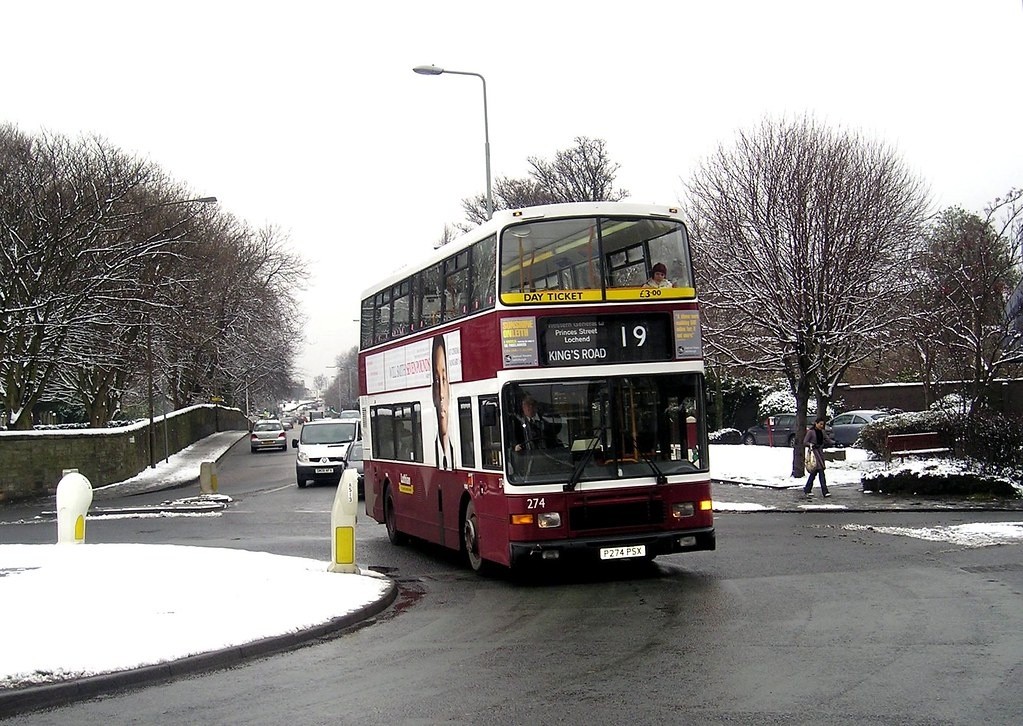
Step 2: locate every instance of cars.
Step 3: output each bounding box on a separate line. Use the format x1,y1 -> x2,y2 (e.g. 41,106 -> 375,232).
825,410 -> 890,446
250,420 -> 288,453
340,409 -> 359,421
344,441 -> 363,496
283,410 -> 307,428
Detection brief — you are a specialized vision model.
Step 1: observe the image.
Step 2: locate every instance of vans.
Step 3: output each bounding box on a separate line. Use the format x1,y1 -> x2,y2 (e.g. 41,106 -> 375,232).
292,419 -> 359,487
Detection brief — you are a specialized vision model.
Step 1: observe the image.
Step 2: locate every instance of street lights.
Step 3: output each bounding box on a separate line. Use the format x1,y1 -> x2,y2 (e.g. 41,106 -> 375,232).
413,65 -> 492,221
143,196 -> 218,468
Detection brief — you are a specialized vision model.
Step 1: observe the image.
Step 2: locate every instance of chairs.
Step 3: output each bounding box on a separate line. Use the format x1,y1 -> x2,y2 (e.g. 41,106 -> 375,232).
374,294 -> 495,344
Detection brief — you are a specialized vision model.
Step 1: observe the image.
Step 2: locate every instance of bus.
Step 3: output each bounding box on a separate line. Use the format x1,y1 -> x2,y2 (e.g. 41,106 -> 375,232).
358,202 -> 716,573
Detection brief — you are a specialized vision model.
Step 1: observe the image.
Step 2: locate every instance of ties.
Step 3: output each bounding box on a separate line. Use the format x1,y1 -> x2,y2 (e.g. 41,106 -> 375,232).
530,417 -> 533,423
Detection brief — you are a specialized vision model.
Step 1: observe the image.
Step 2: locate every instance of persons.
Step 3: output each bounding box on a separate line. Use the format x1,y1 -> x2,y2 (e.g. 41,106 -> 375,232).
515,396 -> 563,450
642,262 -> 673,287
803,418 -> 831,496
431,335 -> 458,467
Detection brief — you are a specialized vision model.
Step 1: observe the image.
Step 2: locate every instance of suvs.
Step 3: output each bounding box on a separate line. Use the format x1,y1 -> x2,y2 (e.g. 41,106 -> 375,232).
742,413 -> 826,447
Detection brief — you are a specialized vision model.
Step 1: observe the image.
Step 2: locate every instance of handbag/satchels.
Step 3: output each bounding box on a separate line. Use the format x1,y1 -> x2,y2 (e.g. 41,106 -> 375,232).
806,443 -> 817,473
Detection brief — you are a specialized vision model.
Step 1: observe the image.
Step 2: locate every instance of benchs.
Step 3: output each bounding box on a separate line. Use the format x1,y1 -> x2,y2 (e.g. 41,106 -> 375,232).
885,431 -> 955,465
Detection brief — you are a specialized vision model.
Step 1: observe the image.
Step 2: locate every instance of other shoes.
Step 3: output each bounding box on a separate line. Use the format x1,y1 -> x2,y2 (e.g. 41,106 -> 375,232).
825,493 -> 831,497
806,492 -> 815,496
